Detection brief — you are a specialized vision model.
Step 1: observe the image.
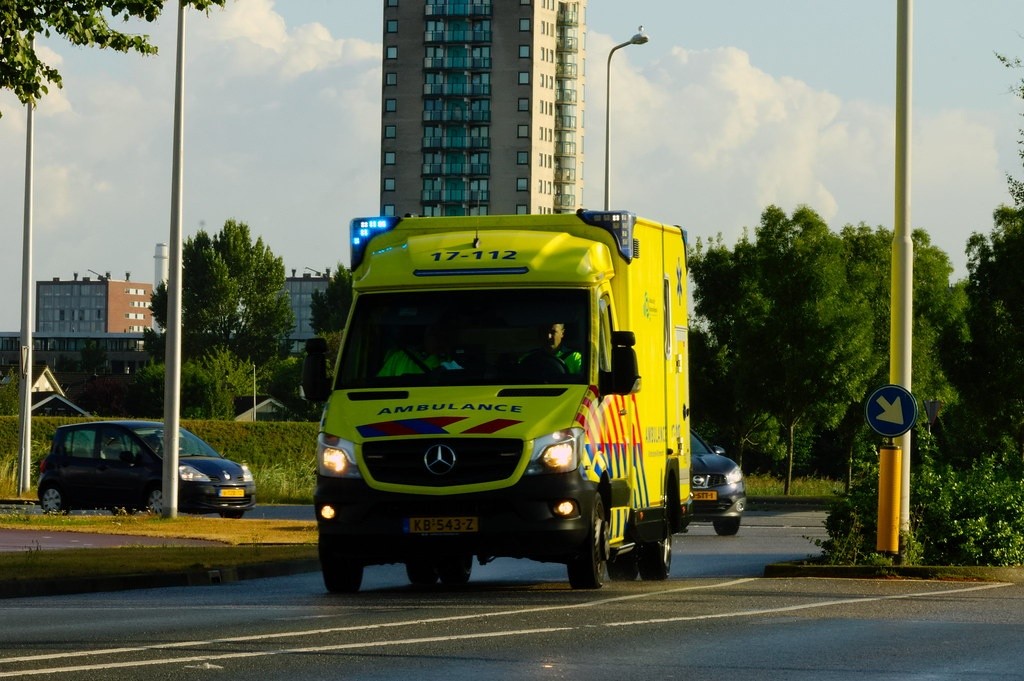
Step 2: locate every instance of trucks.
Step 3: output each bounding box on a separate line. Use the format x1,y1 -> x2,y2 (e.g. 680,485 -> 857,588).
313,208 -> 692,589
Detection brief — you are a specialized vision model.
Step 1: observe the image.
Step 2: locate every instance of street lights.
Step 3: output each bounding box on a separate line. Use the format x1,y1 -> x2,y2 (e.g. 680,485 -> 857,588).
606,35 -> 651,211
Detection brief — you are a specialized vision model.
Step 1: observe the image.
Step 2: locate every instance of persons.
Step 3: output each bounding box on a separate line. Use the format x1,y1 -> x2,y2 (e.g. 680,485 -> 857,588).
510,315 -> 583,380
136,434 -> 162,466
374,325 -> 467,375
179,432 -> 188,453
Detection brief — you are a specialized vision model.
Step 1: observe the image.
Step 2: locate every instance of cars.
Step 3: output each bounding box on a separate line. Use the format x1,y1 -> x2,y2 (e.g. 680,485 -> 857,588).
36,420 -> 257,518
675,428 -> 746,535
